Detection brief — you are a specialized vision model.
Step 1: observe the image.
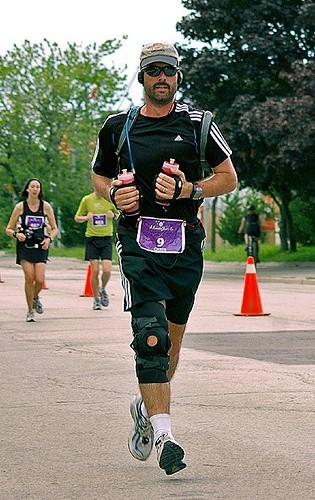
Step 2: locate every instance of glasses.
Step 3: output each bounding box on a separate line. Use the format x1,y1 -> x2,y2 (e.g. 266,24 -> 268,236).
144,66 -> 177,76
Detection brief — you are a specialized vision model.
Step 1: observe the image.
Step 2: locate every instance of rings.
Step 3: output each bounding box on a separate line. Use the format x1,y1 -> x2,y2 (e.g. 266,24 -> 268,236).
163,188 -> 166,192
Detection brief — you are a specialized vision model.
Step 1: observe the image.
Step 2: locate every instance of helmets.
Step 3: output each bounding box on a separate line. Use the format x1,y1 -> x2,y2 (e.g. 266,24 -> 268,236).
246,205 -> 255,210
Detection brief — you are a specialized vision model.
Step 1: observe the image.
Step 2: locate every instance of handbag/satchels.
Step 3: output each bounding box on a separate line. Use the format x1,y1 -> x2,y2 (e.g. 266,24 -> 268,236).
23,243 -> 43,262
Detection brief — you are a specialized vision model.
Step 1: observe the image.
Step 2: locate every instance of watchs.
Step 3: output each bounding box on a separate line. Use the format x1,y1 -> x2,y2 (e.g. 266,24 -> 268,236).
190,182 -> 203,200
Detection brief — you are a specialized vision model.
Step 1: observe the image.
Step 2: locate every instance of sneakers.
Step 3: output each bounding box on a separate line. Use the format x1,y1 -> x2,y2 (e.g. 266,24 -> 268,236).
32,299 -> 43,314
93,298 -> 101,310
27,312 -> 36,322
99,289 -> 109,306
127,397 -> 155,461
157,439 -> 186,476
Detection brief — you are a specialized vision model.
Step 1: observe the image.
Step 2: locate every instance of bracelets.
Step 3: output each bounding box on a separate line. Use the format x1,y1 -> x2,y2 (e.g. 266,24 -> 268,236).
49,237 -> 52,243
13,232 -> 17,238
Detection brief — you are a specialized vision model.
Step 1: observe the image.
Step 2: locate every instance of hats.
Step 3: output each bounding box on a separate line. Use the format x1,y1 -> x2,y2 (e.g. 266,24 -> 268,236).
140,42 -> 179,67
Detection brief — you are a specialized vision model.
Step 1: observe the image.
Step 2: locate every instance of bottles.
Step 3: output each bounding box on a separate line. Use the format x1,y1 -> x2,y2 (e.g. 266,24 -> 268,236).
117,168 -> 140,216
155,158 -> 179,206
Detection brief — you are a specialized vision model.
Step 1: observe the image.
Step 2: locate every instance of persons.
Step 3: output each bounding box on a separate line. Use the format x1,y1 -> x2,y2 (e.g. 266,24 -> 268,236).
238,204 -> 260,250
6,179 -> 58,322
92,41 -> 237,475
75,186 -> 120,310
42,217 -> 52,288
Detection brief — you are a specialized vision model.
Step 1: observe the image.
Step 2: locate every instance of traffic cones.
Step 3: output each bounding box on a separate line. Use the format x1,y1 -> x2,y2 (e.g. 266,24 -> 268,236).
80,264 -> 102,298
231,254 -> 273,318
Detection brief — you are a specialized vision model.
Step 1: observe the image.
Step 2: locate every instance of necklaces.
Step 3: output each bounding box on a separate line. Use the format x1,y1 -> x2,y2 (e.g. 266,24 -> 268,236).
168,102 -> 174,113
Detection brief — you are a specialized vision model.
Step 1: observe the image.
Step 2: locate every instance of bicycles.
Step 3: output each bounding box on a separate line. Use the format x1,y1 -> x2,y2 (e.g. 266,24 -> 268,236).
247,240 -> 258,264
